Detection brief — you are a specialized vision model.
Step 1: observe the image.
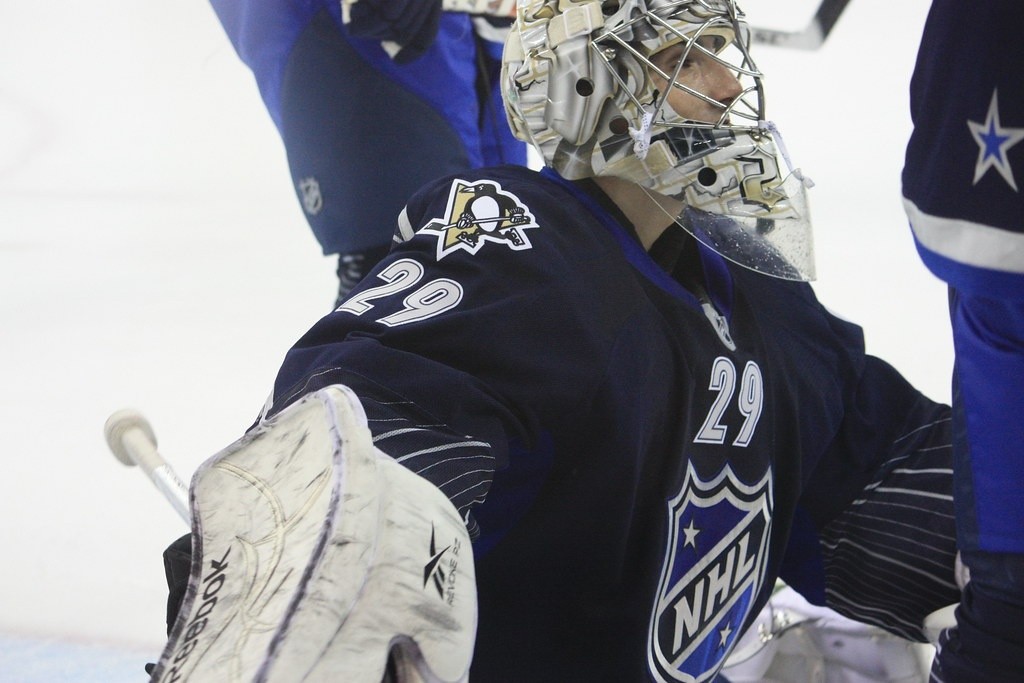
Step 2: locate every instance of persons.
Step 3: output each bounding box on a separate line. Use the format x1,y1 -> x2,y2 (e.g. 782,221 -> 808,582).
153,0 -> 1024,683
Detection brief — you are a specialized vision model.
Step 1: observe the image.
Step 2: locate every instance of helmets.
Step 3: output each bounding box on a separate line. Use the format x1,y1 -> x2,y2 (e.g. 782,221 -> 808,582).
500,1 -> 802,225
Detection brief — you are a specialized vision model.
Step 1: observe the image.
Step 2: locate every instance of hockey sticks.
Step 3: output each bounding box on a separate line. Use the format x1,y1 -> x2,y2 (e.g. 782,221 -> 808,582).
106,410 -> 440,682
746,0 -> 852,53
